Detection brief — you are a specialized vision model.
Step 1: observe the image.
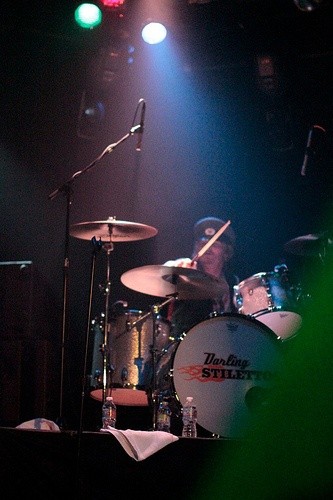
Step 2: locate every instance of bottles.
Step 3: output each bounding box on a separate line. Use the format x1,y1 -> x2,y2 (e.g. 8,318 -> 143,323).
102,396 -> 117,430
182,396 -> 198,438
157,402 -> 171,434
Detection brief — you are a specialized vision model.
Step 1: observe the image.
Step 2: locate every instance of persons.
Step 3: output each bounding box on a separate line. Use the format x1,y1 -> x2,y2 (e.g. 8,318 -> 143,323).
147,218 -> 244,428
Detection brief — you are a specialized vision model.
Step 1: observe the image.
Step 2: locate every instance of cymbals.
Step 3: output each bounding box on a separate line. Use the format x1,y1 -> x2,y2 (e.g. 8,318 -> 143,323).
284,236 -> 331,257
69,219 -> 158,242
120,264 -> 229,300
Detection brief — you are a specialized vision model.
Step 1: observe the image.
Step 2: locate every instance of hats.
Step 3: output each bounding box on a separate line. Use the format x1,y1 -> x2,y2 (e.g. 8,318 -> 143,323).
194,217 -> 239,252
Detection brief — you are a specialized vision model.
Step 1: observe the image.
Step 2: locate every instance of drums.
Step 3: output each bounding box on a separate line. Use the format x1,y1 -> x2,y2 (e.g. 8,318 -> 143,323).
154,311 -> 285,439
232,271 -> 305,340
87,310 -> 174,406
